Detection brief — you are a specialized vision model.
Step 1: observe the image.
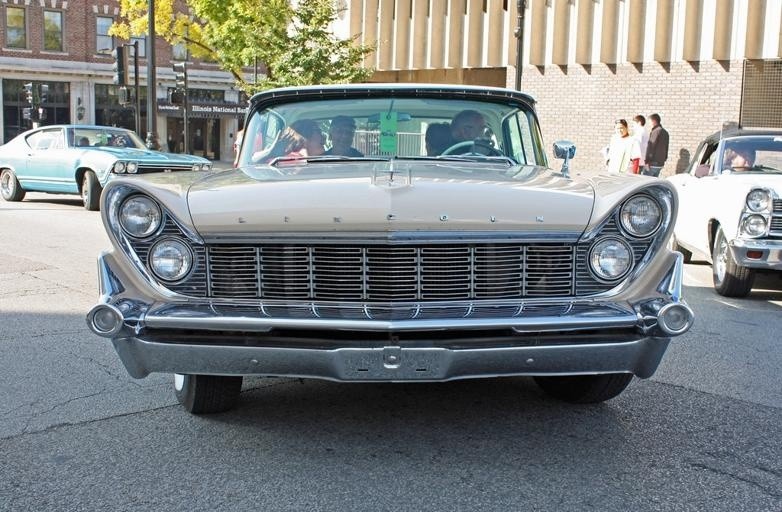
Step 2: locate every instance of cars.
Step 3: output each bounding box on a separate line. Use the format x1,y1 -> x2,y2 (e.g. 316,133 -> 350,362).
84,82 -> 695,415
667,122 -> 782,296
0,124 -> 214,211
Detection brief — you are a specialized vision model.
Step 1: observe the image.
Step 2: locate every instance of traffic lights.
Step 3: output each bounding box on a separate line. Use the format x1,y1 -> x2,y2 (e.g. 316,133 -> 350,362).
111,46 -> 124,85
170,92 -> 185,106
119,88 -> 127,105
173,63 -> 186,90
39,84 -> 49,104
21,84 -> 33,103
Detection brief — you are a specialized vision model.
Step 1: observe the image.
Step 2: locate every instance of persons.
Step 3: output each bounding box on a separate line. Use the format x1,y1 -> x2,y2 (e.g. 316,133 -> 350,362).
693,146 -> 762,180
106,135 -> 120,147
426,122 -> 451,157
605,112 -> 670,178
440,109 -> 521,165
234,111 -> 364,169
79,137 -> 90,146
168,135 -> 176,153
179,130 -> 186,152
146,133 -> 160,150
